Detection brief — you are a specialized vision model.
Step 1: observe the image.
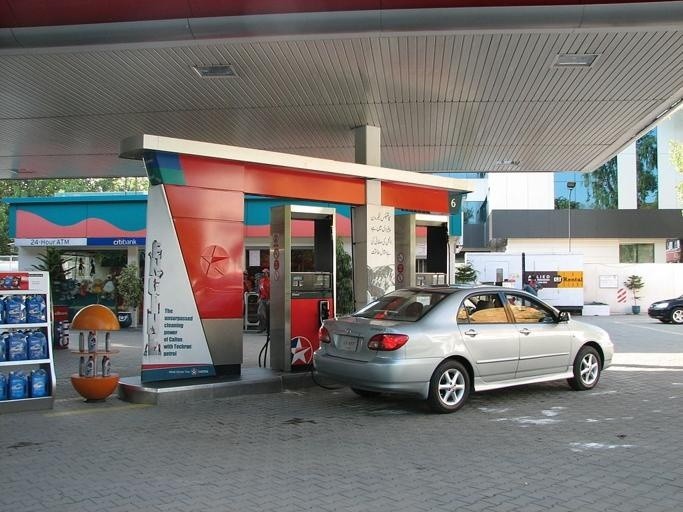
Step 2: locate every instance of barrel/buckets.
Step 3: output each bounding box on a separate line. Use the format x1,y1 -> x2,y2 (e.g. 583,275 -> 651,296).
0,294 -> 50,400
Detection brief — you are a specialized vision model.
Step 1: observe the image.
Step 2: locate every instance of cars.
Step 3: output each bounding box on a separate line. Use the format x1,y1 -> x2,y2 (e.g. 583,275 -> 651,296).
312,283 -> 615,412
647,295 -> 682,324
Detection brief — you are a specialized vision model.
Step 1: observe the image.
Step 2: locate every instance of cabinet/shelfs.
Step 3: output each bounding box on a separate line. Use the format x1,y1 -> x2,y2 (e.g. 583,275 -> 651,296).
0,273 -> 123,415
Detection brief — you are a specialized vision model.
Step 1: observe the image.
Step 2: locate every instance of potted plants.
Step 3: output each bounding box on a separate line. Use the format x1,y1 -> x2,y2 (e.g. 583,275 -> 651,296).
624,274 -> 644,316
115,262 -> 144,328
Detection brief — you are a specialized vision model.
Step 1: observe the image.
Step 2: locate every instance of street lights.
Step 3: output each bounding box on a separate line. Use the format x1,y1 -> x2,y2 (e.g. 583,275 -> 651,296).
565,181 -> 576,252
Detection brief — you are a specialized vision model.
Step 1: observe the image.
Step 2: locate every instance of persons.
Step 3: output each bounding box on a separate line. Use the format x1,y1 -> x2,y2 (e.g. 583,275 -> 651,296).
253,273 -> 270,333
261,268 -> 269,279
527,274 -> 541,308
241,269 -> 252,330
